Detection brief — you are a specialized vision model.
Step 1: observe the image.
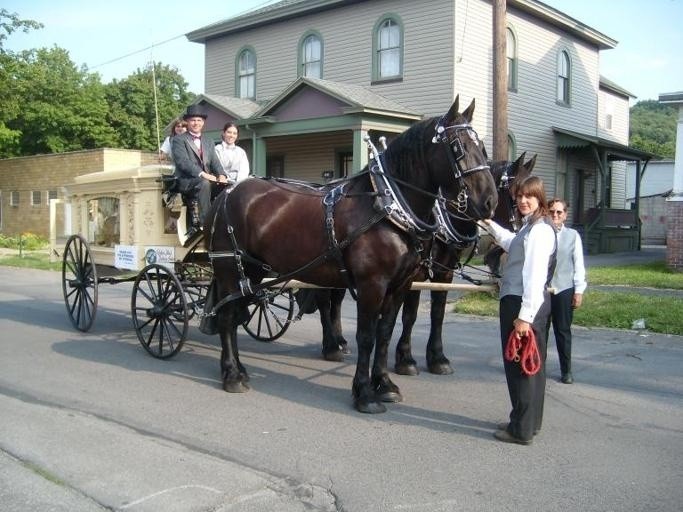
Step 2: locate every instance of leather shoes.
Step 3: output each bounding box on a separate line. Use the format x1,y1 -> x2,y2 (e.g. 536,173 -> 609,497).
561,371 -> 573,383
494,423 -> 534,445
497,421 -> 540,435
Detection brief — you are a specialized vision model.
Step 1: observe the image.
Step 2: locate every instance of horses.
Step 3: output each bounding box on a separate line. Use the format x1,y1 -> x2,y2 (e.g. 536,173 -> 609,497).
312,151 -> 538,376
202,92 -> 498,413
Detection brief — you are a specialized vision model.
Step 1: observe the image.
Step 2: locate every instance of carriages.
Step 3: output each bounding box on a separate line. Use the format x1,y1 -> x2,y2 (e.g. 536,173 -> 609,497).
60,58 -> 558,418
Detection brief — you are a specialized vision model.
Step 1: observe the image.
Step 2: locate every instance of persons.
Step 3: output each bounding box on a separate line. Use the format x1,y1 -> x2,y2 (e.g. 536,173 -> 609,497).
160,120 -> 191,173
173,104 -> 230,235
212,121 -> 251,185
547,199 -> 587,382
474,176 -> 557,446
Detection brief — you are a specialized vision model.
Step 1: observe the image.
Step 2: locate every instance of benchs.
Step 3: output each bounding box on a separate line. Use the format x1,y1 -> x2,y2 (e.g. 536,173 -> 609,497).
160,174 -> 202,240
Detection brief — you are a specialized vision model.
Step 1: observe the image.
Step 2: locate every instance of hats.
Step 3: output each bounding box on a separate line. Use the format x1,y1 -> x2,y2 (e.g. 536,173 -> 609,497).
183,105 -> 208,120
